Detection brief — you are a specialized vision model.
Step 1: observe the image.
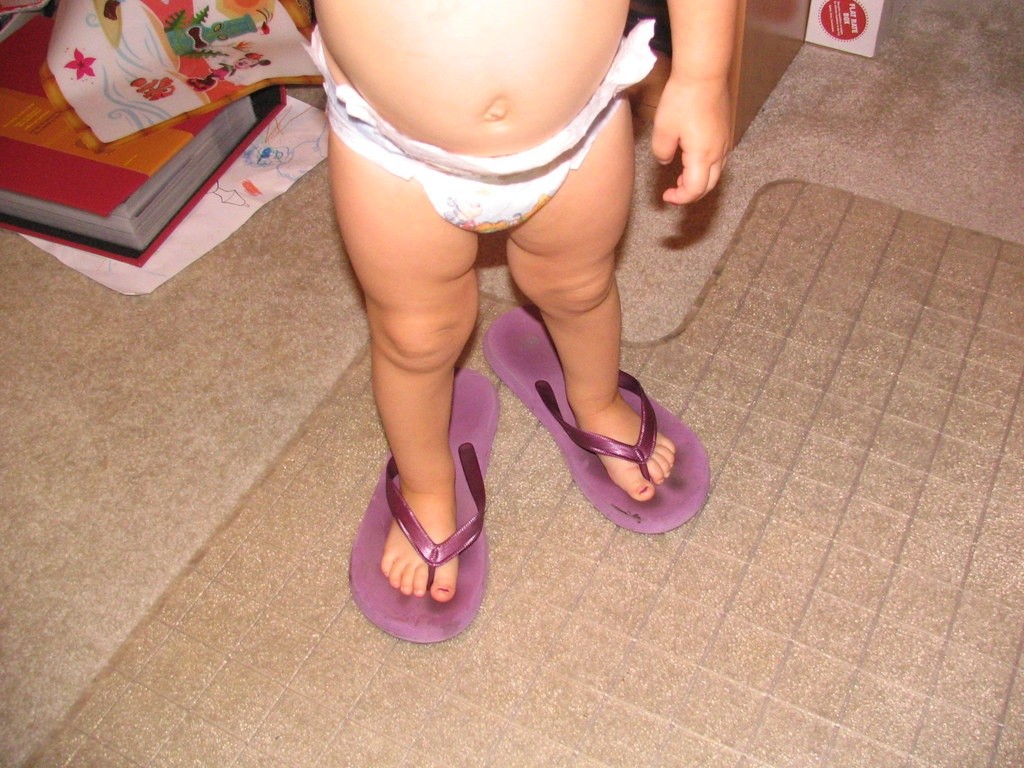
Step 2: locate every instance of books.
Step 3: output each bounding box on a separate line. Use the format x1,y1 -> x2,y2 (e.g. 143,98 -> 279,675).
0,11 -> 287,268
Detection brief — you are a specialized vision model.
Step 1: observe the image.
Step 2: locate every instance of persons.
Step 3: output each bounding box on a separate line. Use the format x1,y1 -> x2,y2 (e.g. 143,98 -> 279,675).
311,0 -> 739,606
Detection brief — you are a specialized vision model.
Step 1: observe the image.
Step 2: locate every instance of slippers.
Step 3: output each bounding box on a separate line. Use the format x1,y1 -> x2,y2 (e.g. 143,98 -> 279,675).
482,305 -> 711,535
349,366 -> 500,644
